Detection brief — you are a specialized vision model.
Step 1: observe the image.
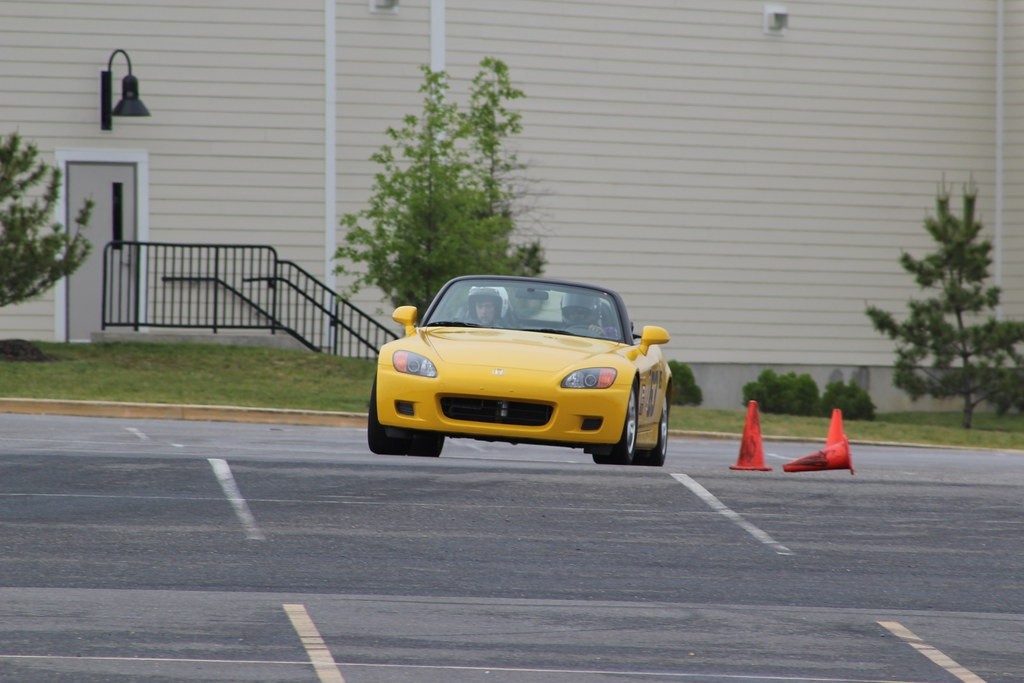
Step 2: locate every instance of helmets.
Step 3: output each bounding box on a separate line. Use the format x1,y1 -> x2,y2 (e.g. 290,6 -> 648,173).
560,291 -> 601,327
468,286 -> 509,318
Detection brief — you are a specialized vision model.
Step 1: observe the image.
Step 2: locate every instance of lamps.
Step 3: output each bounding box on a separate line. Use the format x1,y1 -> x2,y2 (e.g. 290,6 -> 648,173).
100,49 -> 151,132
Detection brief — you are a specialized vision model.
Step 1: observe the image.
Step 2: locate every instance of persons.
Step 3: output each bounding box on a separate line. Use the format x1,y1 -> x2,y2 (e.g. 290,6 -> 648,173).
467,286 -> 504,326
560,293 -> 608,338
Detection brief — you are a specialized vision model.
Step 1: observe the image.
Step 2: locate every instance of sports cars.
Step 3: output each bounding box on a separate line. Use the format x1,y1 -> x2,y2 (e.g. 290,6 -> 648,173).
367,275 -> 674,466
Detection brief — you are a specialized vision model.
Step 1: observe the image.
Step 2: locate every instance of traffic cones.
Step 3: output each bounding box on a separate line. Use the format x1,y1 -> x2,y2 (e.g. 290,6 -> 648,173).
728,401 -> 773,472
784,435 -> 855,476
826,409 -> 852,458
